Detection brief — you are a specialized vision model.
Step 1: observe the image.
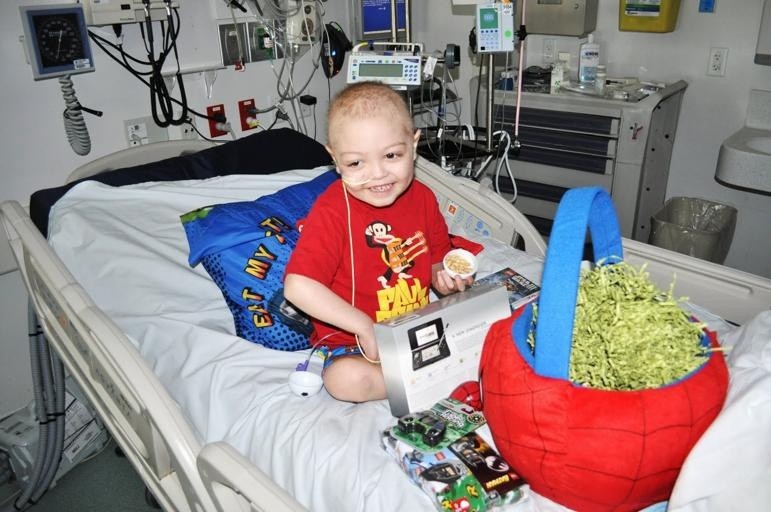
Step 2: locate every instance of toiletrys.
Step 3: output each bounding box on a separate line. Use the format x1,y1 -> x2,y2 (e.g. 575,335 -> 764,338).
578,32 -> 600,84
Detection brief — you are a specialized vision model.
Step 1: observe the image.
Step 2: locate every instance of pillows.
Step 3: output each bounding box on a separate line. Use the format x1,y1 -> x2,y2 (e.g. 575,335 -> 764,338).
178,168 -> 342,353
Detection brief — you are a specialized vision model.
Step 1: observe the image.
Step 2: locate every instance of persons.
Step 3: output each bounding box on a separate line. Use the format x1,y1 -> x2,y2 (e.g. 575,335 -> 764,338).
282,81 -> 474,403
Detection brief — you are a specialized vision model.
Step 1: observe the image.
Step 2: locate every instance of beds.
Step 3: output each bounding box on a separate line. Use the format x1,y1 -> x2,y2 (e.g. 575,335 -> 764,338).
1,127 -> 770,512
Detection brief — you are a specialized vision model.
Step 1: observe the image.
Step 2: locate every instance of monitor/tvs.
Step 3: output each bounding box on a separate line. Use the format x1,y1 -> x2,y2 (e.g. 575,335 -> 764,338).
356,0 -> 406,41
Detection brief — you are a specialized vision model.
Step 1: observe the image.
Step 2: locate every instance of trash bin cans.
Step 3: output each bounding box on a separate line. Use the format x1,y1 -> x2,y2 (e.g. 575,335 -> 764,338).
648,196 -> 738,265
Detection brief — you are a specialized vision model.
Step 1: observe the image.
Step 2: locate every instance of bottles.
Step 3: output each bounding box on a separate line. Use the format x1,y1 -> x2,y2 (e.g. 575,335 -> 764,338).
551,61 -> 564,93
595,64 -> 608,96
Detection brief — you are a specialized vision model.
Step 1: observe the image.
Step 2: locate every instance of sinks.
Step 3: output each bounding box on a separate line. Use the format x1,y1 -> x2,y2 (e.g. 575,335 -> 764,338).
745,135 -> 771,156
